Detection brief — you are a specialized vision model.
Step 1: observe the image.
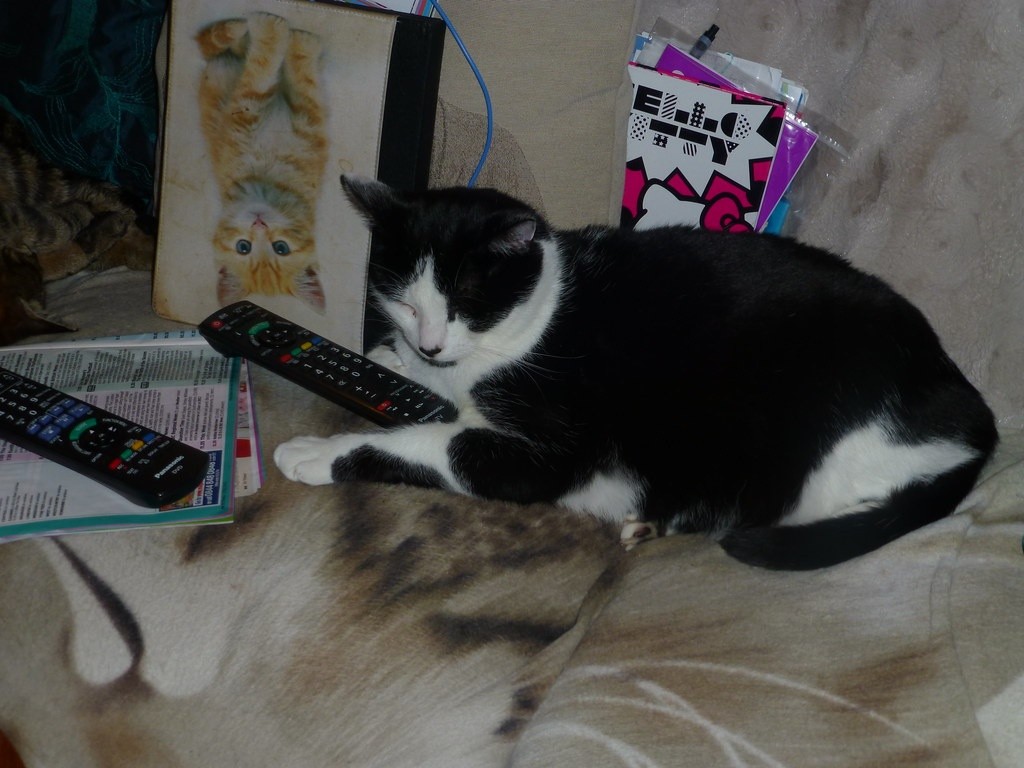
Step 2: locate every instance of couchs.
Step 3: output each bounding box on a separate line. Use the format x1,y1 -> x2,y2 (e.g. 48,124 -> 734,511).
0,0 -> 1024,768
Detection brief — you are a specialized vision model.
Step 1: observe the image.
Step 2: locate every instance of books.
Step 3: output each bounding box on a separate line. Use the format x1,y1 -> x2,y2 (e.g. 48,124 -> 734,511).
1,329 -> 266,546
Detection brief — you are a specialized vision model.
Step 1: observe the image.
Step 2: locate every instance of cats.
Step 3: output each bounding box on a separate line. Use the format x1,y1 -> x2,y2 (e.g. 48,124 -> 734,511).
271,176 -> 1000,571
193,13 -> 328,314
1,110 -> 157,346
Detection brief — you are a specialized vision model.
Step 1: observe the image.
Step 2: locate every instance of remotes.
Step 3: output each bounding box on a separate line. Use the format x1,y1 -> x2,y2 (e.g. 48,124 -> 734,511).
0,365 -> 208,510
198,301 -> 459,429
